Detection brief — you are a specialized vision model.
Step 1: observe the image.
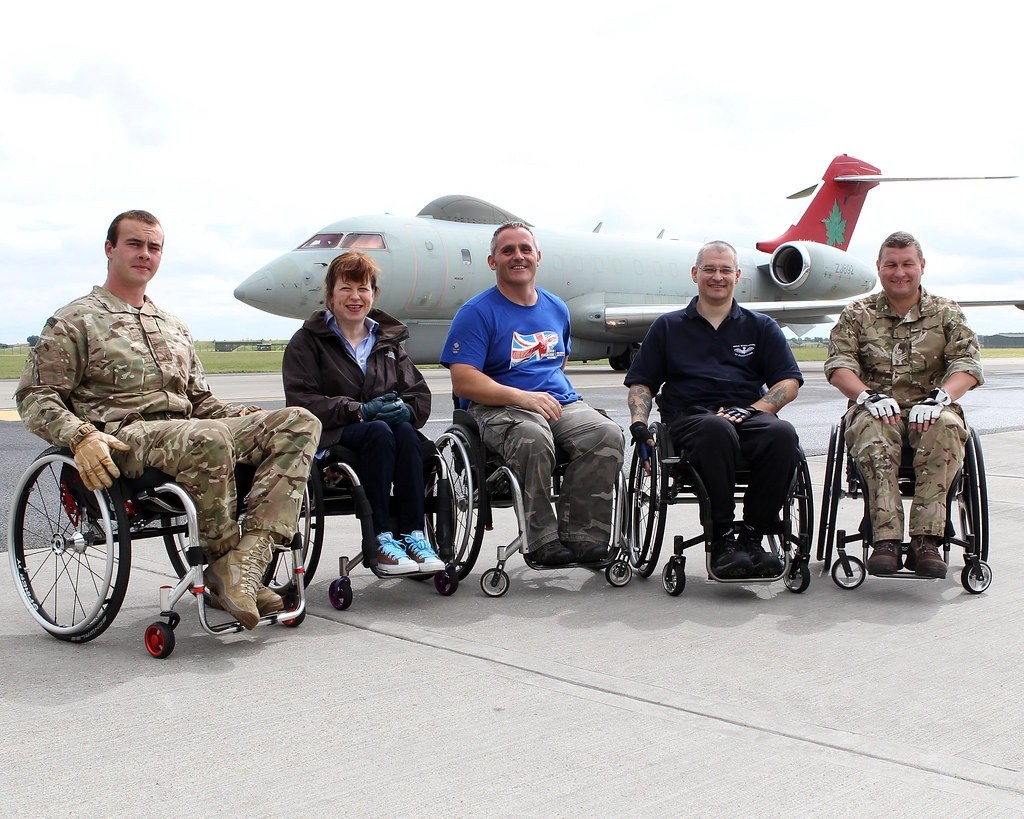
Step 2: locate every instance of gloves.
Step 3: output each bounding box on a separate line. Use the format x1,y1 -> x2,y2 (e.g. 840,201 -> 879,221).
372,396 -> 409,423
362,389 -> 400,422
70,425 -> 130,491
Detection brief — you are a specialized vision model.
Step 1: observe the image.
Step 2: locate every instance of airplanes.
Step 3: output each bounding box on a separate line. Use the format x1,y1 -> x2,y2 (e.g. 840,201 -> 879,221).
231,153 -> 1024,373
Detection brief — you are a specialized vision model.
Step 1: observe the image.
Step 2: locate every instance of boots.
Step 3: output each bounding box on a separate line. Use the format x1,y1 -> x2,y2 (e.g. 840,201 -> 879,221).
205,551 -> 283,614
203,529 -> 277,631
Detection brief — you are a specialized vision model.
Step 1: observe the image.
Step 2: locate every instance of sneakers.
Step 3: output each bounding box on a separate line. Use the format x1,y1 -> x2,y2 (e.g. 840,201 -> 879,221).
375,533 -> 418,574
739,533 -> 784,578
713,532 -> 754,580
534,538 -> 571,565
868,540 -> 903,575
903,535 -> 948,580
564,538 -> 607,563
401,531 -> 446,571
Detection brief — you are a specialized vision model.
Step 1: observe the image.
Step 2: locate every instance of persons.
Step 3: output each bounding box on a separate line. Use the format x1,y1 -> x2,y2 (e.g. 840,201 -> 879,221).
11,210 -> 322,626
437,222 -> 624,567
624,240 -> 805,584
825,230 -> 985,578
283,250 -> 446,575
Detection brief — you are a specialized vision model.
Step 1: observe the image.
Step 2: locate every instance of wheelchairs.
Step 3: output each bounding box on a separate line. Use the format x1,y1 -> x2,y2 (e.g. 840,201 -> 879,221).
7,445 -> 308,660
419,388 -> 633,600
620,420 -> 813,598
816,395 -> 994,595
263,422 -> 474,611
